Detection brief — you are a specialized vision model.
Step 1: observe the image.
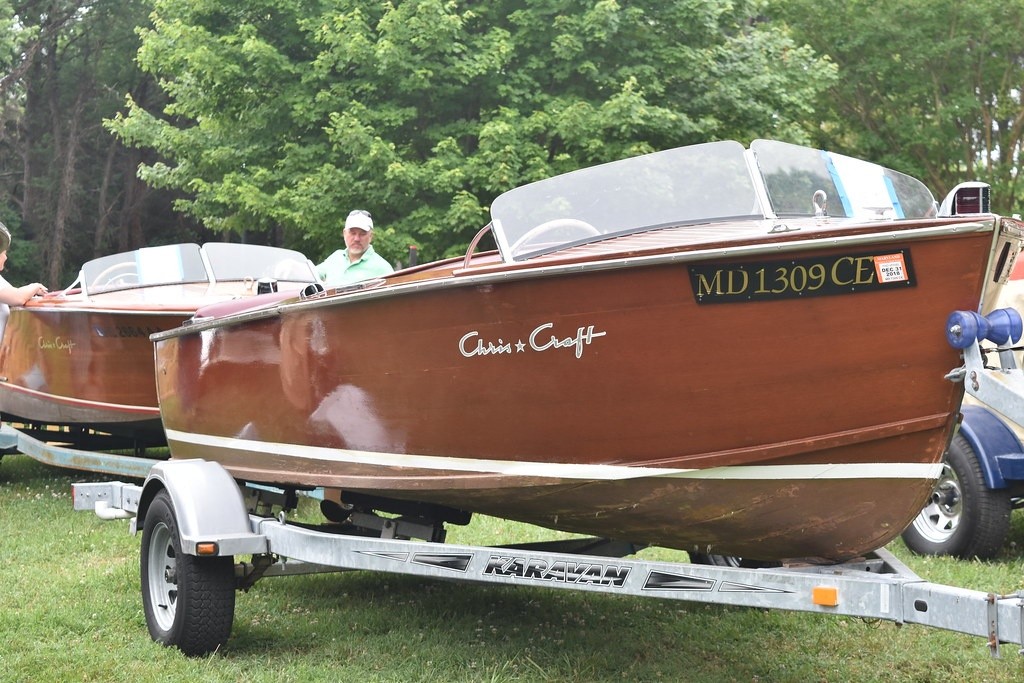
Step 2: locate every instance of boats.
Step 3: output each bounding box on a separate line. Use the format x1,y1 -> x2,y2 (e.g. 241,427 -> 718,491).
2,238 -> 327,442
987,225 -> 1024,344
152,136 -> 1023,559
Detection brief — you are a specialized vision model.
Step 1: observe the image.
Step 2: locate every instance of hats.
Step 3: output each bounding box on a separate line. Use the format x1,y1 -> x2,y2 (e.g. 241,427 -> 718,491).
345,211 -> 373,232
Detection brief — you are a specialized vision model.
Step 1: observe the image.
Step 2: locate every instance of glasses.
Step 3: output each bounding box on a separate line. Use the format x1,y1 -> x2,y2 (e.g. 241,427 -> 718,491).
347,210 -> 372,219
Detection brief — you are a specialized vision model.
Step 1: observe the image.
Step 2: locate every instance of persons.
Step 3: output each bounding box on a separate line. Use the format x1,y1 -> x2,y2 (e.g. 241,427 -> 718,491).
0,221 -> 48,345
287,211 -> 394,524
